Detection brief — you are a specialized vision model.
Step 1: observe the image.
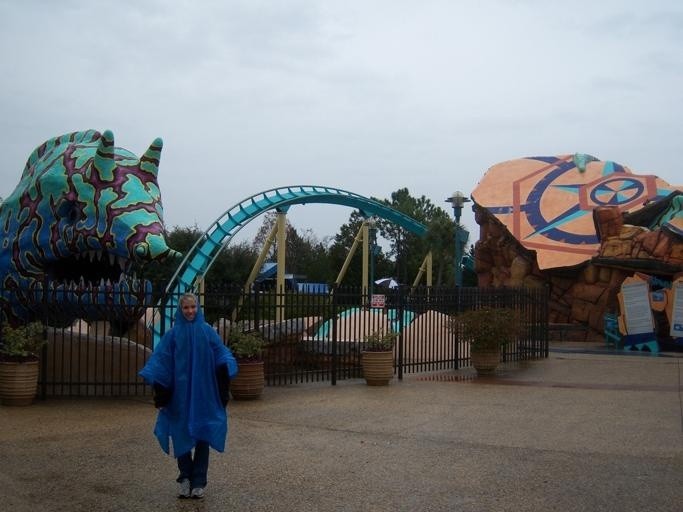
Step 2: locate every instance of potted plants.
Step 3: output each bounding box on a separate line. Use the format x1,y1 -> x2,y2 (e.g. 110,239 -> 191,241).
358,326 -> 402,386
1,321 -> 50,409
444,307 -> 525,377
227,323 -> 303,402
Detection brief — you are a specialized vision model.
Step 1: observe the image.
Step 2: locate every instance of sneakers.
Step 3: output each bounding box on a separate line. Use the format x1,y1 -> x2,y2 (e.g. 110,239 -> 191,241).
191,488 -> 204,498
178,478 -> 190,498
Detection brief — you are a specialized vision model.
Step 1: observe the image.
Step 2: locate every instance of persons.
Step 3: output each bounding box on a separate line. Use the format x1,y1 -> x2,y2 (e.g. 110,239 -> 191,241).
138,291 -> 242,498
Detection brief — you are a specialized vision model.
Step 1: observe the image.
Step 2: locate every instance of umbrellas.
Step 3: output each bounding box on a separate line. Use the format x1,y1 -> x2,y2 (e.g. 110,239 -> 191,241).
374,276 -> 399,289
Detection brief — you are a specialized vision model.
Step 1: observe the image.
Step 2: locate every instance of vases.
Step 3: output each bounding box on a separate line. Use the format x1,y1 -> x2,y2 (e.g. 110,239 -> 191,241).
319,353 -> 358,380
